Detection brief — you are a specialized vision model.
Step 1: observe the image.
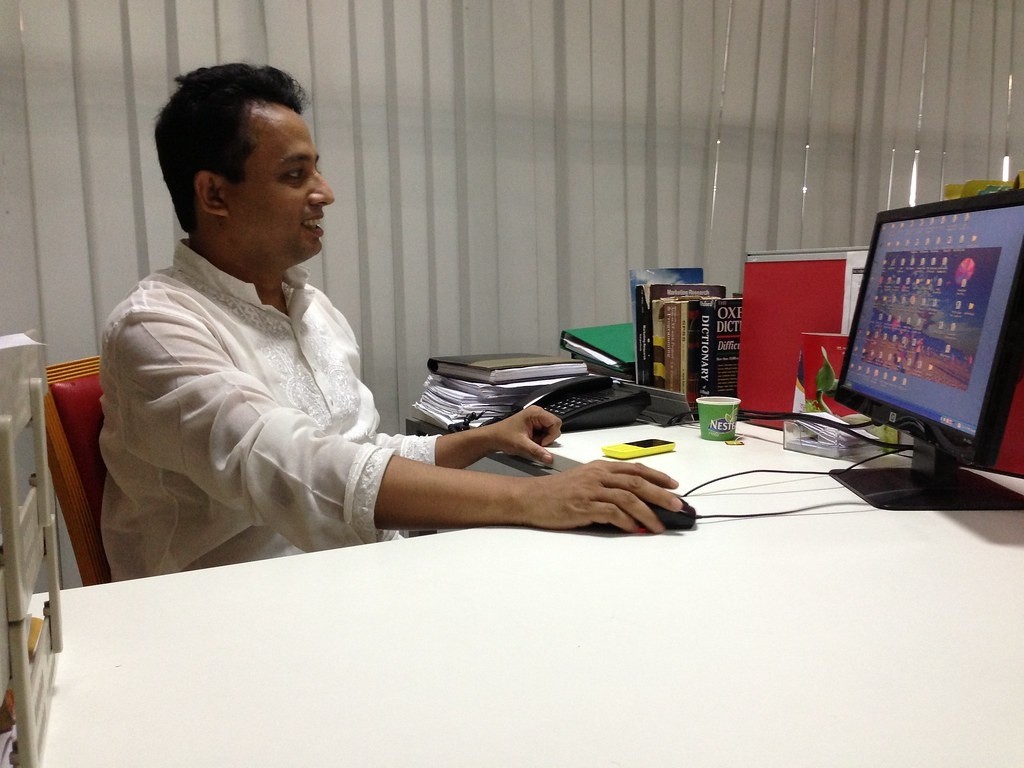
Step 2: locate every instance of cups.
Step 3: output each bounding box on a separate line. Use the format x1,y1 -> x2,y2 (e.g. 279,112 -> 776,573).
696,397 -> 741,441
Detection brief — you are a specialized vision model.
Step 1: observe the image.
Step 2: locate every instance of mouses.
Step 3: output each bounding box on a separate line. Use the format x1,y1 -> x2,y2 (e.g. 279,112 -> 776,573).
595,497 -> 698,533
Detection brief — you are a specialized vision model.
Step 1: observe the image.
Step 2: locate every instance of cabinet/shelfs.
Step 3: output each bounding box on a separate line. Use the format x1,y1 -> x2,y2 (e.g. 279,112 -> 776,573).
0,333 -> 61,768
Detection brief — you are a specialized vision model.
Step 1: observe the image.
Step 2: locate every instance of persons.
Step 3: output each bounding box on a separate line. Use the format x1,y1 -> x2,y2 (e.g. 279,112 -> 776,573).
100,63 -> 681,584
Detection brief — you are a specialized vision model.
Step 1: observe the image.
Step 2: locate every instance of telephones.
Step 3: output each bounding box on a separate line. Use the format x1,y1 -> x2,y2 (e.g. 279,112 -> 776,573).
511,374 -> 653,432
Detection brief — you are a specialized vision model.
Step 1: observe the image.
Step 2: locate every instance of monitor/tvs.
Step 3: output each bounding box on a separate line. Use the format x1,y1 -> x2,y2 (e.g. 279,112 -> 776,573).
832,188 -> 1024,468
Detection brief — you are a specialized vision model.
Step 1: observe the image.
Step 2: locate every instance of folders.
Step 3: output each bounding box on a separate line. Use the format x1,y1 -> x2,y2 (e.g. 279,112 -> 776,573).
558,321 -> 637,374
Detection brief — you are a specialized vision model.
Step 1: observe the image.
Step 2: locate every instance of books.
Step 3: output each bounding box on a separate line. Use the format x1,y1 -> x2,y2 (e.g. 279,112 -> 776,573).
427,353 -> 589,385
629,267 -> 743,421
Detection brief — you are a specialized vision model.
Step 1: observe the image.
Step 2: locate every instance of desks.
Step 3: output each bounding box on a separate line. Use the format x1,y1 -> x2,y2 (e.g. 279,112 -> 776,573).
31,421 -> 1024,768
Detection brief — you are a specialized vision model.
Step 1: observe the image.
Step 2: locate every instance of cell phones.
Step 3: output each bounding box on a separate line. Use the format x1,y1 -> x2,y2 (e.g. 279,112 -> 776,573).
602,440 -> 676,460
